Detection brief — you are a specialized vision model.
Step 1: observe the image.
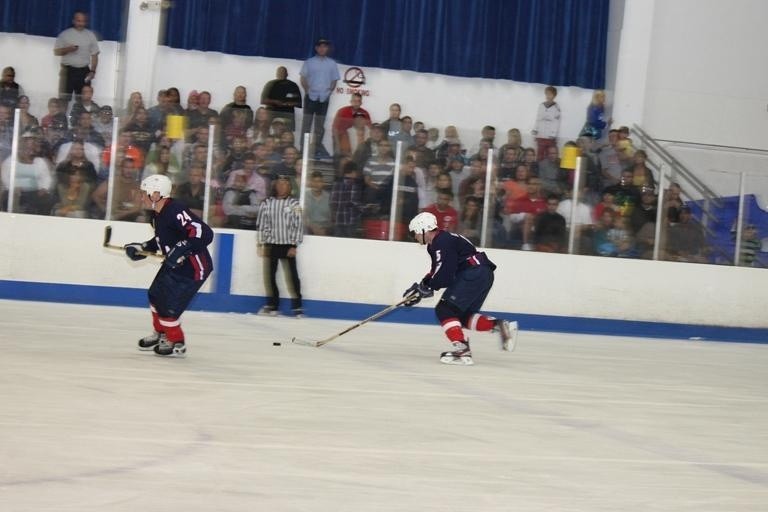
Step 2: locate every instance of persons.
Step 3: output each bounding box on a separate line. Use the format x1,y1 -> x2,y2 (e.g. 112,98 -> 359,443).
296,38 -> 342,144
126,174 -> 218,360
400,212 -> 518,364
731,216 -> 762,267
3,66 -> 708,264
251,176 -> 308,319
52,11 -> 101,103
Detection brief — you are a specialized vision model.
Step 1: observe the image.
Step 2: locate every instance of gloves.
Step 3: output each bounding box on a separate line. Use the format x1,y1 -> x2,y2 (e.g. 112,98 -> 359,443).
401,282 -> 421,308
417,280 -> 435,300
162,240 -> 192,270
125,235 -> 157,261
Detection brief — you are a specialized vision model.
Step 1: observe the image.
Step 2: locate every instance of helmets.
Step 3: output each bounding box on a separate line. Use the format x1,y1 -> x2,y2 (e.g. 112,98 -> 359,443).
408,211 -> 439,237
139,174 -> 174,198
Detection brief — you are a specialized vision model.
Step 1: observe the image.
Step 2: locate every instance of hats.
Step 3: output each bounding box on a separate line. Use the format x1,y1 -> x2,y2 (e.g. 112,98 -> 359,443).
313,36 -> 331,46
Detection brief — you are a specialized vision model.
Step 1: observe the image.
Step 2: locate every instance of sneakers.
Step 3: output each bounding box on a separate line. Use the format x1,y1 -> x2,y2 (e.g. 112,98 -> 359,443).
259,303 -> 279,312
293,308 -> 304,317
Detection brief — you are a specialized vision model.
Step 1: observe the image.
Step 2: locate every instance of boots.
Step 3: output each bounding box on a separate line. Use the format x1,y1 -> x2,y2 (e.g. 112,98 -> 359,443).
154,337 -> 186,355
139,330 -> 167,348
440,337 -> 473,358
492,317 -> 511,352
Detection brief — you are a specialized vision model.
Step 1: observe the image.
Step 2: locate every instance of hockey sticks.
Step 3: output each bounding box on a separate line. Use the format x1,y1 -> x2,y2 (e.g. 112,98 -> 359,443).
104,225 -> 167,260
292,292 -> 421,348
